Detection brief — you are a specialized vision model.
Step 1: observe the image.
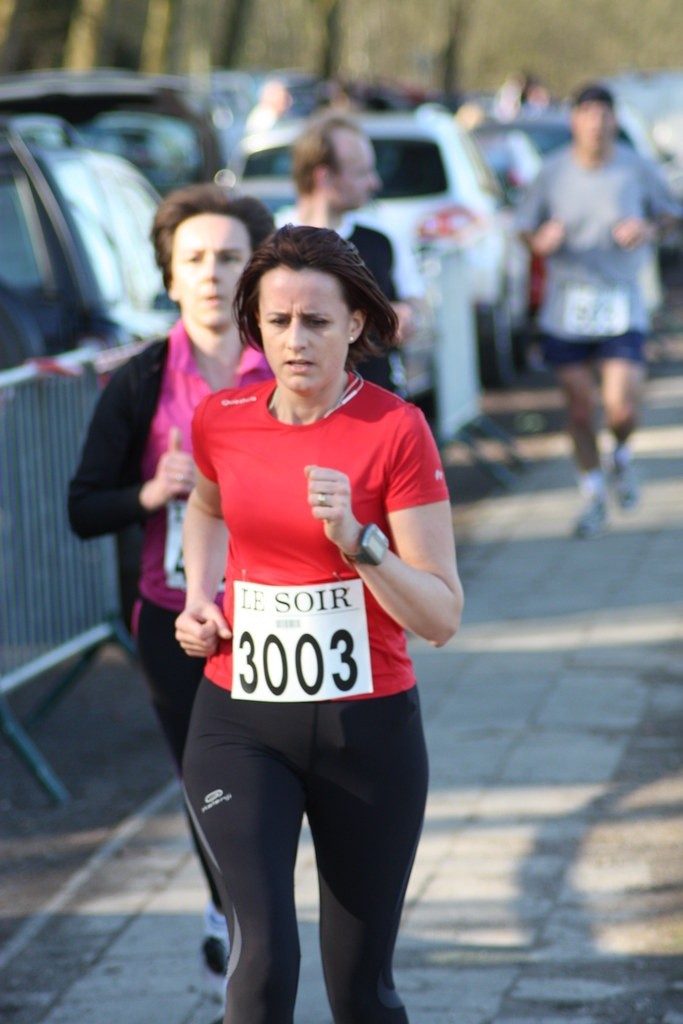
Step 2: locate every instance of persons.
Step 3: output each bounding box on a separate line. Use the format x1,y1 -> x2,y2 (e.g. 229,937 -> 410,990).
69,183 -> 275,1001
277,119 -> 418,406
237,76 -> 558,188
515,84 -> 683,535
176,226 -> 466,1023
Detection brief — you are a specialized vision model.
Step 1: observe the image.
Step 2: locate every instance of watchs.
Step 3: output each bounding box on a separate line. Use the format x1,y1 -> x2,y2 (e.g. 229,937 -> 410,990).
339,522 -> 388,571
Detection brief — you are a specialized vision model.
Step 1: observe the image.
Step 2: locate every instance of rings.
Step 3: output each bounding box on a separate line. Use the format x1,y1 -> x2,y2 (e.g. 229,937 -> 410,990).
318,494 -> 327,507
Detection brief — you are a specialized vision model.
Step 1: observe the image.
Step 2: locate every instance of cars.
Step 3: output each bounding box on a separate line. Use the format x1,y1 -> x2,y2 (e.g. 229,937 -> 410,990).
0,64 -> 683,648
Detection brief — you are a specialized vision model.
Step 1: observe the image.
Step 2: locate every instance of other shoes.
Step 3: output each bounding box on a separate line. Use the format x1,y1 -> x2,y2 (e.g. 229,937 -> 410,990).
574,500 -> 612,540
199,927 -> 232,994
614,450 -> 639,509
